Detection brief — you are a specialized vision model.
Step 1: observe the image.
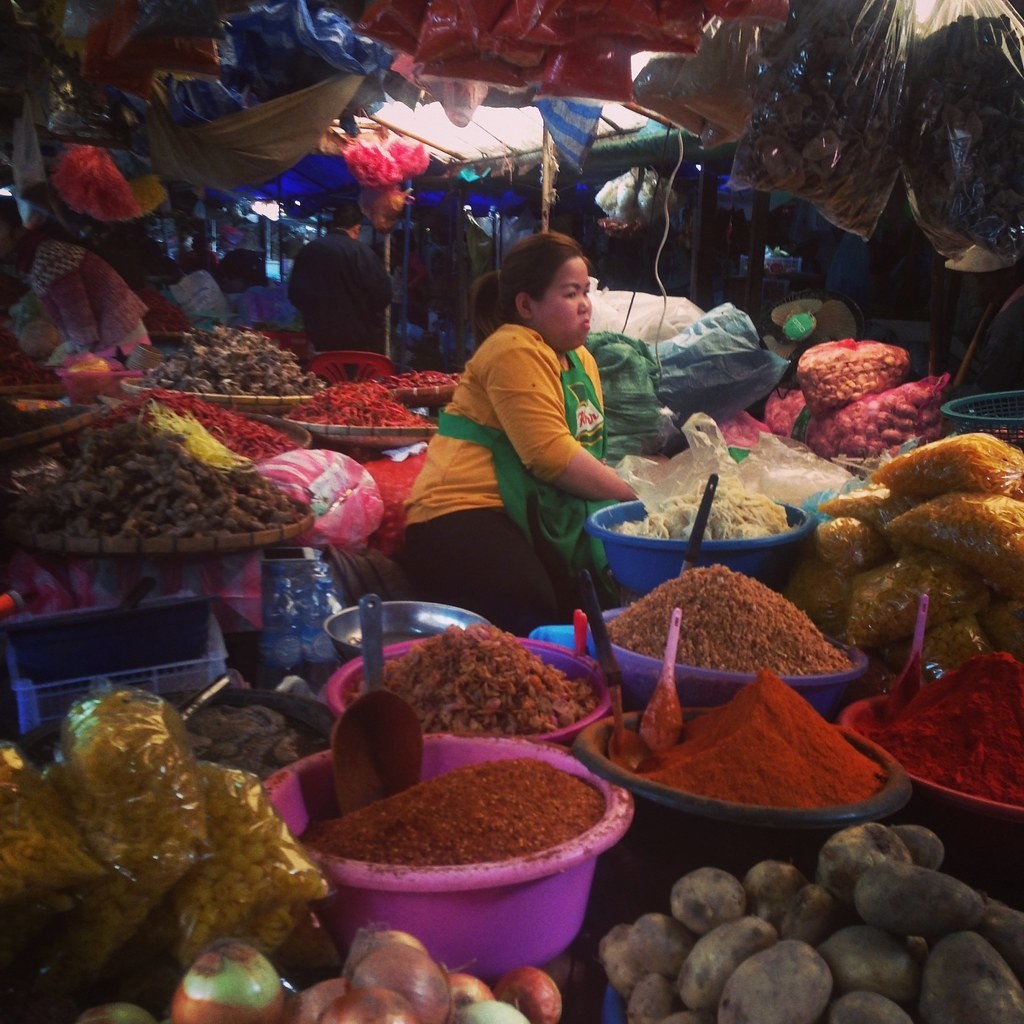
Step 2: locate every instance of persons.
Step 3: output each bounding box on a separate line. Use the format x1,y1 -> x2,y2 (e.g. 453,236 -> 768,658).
404,234 -> 638,639
288,204 -> 430,355
84,220 -> 217,297
928,256 -> 1024,414
0,199 -> 152,363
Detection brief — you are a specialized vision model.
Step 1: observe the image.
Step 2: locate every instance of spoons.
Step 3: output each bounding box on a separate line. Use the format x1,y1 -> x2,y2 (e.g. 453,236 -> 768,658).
884,594 -> 928,717
640,608 -> 681,750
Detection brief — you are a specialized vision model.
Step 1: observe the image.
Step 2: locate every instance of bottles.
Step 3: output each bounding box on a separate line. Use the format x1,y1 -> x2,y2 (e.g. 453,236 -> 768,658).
298,578 -> 342,664
262,579 -> 300,673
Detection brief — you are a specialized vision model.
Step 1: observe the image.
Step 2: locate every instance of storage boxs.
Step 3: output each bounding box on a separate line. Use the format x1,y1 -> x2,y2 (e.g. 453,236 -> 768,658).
2,597 -> 231,736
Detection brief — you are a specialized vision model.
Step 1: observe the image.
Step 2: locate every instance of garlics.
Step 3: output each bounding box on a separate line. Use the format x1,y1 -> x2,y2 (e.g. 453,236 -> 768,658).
764,373 -> 945,458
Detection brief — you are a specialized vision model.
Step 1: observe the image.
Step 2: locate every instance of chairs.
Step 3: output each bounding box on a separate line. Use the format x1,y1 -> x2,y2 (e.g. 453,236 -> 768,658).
302,350 -> 397,388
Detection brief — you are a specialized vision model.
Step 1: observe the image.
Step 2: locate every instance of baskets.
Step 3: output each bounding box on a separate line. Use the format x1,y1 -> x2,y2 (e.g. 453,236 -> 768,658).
939,390 -> 1024,452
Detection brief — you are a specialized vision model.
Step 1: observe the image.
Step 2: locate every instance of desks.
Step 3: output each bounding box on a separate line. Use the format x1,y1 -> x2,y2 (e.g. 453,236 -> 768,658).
237,324 -> 311,362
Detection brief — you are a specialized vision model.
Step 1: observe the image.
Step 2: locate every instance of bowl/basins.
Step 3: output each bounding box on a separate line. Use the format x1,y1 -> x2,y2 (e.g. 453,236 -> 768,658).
325,640 -> 612,746
572,710 -> 911,903
582,604 -> 870,721
57,368 -> 144,406
839,694 -> 1024,876
263,732 -> 635,984
583,501 -> 812,599
325,601 -> 491,663
603,981 -> 627,1024
17,687 -> 335,784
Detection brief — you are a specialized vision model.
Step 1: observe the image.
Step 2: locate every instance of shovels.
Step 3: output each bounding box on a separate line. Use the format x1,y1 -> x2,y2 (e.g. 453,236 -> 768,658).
577,569 -> 647,769
331,594 -> 425,818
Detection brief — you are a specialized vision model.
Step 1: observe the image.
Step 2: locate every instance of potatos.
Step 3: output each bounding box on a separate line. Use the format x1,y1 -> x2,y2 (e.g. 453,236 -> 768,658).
598,824 -> 1024,1023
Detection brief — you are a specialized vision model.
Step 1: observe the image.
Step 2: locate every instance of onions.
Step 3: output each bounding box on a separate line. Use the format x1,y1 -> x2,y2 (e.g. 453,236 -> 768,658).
72,927 -> 565,1024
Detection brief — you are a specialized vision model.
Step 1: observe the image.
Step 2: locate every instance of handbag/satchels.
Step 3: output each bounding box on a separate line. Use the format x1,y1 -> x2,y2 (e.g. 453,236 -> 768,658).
615,412 -> 744,514
352,0 -> 1024,272
